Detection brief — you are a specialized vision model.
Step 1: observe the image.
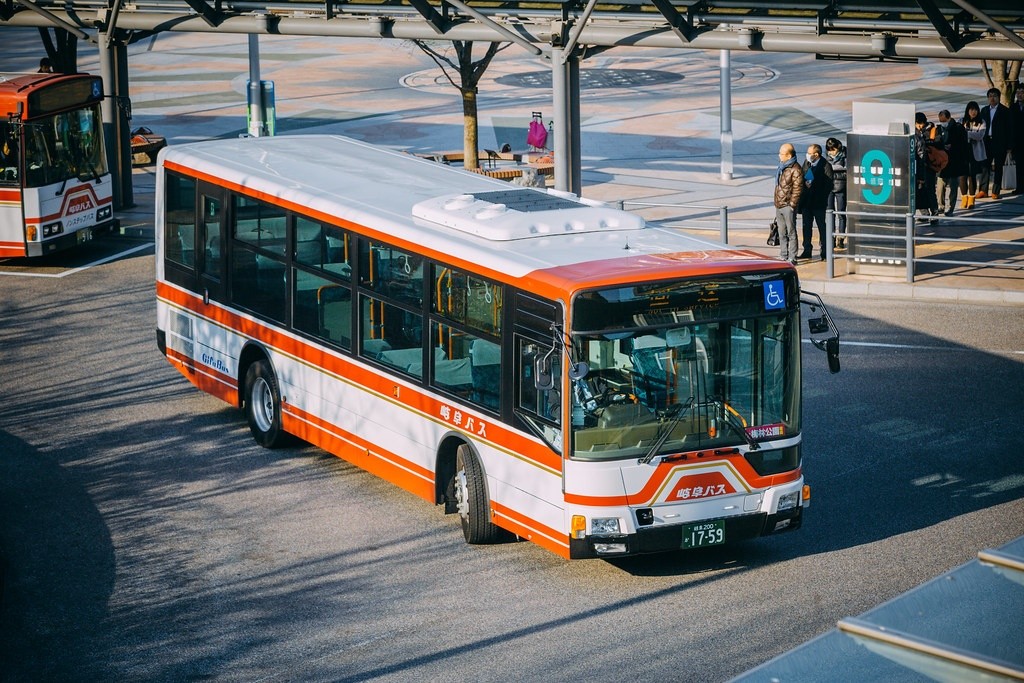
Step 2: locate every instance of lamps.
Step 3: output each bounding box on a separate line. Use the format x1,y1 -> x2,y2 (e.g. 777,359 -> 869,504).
738,30 -> 752,46
255,16 -> 267,30
871,35 -> 886,51
369,18 -> 384,33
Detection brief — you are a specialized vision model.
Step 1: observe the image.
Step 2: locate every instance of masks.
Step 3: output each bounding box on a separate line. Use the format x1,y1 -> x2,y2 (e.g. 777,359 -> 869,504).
806,154 -> 816,161
940,119 -> 949,127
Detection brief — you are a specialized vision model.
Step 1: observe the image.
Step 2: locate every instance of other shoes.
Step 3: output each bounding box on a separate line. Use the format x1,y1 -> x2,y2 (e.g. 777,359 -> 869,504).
787,259 -> 797,265
834,237 -> 836,248
821,254 -> 826,260
839,238 -> 844,248
918,213 -> 930,223
797,251 -> 811,258
992,193 -> 1000,199
945,210 -> 953,216
1012,191 -> 1024,195
976,191 -> 988,198
938,209 -> 944,214
930,211 -> 939,225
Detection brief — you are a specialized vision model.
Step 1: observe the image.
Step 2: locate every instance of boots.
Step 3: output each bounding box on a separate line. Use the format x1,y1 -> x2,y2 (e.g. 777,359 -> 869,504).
960,195 -> 967,208
968,195 -> 975,208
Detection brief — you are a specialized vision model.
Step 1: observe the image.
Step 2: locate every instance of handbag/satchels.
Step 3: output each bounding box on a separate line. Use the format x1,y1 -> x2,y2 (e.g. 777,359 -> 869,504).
526,116 -> 547,149
767,218 -> 781,245
1001,154 -> 1016,190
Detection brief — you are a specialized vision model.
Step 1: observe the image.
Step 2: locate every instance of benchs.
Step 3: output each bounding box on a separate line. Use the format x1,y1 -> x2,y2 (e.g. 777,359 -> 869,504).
131,134 -> 167,168
180,232 -> 501,415
401,150 -> 553,182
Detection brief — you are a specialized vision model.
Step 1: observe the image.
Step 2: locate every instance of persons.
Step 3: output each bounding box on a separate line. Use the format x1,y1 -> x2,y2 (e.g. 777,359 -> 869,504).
797,144 -> 835,262
36,57 -> 53,74
916,83 -> 1024,226
773,143 -> 805,266
0,139 -> 17,181
547,369 -> 603,426
823,138 -> 848,249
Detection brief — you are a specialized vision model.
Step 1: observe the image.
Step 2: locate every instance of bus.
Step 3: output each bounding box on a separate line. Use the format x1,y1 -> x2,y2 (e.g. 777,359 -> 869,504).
154,134 -> 844,561
0,73 -> 131,259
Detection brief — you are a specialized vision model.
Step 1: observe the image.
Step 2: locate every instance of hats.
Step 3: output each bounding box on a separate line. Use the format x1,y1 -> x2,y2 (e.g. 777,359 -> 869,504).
915,112 -> 927,123
